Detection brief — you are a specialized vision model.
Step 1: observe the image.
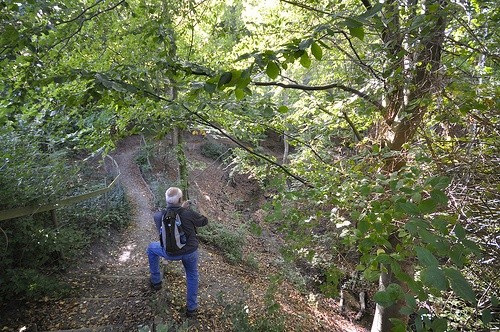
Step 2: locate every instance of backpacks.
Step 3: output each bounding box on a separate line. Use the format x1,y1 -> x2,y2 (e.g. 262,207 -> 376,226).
159,206 -> 187,253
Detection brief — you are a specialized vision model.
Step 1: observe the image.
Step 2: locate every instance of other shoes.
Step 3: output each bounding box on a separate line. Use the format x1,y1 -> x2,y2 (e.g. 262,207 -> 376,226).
185,308 -> 199,316
149,279 -> 162,291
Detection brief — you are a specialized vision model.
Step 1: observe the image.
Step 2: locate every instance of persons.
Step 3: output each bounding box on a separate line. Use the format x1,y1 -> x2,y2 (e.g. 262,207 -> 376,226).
147,186 -> 208,316
